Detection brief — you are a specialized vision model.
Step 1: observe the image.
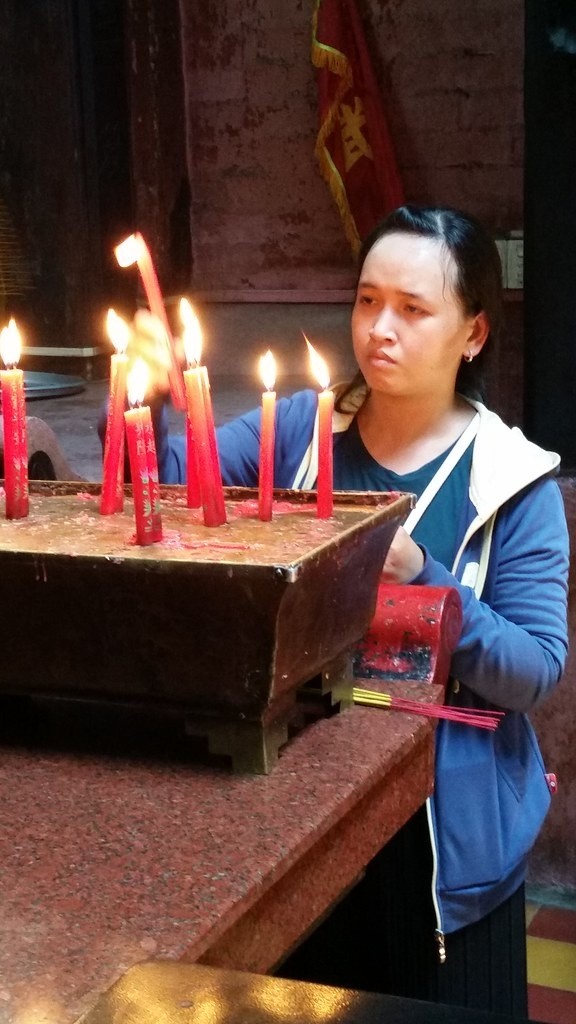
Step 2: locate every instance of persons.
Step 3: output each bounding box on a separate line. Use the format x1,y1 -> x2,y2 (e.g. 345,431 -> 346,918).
98,206 -> 569,1023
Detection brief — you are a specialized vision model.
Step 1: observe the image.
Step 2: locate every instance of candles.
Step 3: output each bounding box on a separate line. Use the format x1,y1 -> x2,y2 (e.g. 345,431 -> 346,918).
186,405 -> 202,509
179,297 -> 226,524
122,366 -> 154,546
97,306 -> 118,516
116,308 -> 129,513
258,349 -> 276,522
302,329 -> 334,519
182,322 -> 223,527
113,231 -> 186,412
8,317 -> 29,518
134,357 -> 163,543
0,325 -> 22,520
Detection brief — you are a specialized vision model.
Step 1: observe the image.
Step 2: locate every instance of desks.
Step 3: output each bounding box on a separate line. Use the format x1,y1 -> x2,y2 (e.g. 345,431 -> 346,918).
73,962 -> 544,1024
0,678 -> 447,1024
